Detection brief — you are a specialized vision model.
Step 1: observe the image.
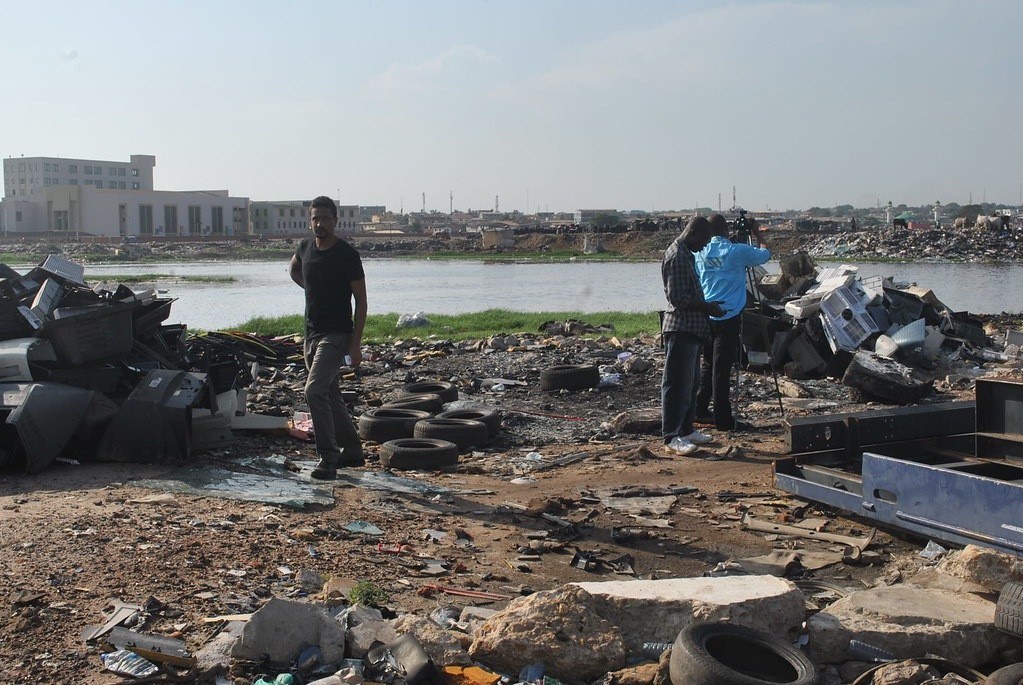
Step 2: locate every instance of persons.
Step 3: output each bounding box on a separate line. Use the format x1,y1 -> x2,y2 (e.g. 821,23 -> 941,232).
692,214 -> 770,430
290,196 -> 366,480
661,217 -> 727,454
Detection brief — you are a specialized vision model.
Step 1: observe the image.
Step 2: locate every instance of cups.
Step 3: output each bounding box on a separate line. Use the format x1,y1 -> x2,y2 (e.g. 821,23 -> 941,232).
347,658 -> 364,676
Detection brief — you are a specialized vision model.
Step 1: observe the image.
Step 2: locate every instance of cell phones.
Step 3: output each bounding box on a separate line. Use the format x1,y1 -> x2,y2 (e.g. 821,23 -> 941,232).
723,308 -> 734,312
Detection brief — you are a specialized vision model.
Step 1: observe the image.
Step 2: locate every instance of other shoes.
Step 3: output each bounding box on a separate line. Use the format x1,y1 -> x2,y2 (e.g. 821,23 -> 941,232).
719,421 -> 745,431
336,451 -> 364,468
311,457 -> 336,479
701,408 -> 712,418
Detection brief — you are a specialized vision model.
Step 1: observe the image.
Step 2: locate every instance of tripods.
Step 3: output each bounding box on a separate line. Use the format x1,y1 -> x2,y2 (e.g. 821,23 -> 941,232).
730,230 -> 785,433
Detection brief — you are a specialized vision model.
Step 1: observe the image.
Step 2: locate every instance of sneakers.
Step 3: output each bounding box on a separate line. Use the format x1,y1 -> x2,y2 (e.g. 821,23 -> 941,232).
681,429 -> 713,444
665,435 -> 697,456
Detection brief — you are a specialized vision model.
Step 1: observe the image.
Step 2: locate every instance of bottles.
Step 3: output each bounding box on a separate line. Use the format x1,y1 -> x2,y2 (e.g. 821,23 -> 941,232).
491,384 -> 504,391
848,639 -> 894,663
100,650 -> 159,678
641,642 -> 672,660
527,452 -> 542,460
